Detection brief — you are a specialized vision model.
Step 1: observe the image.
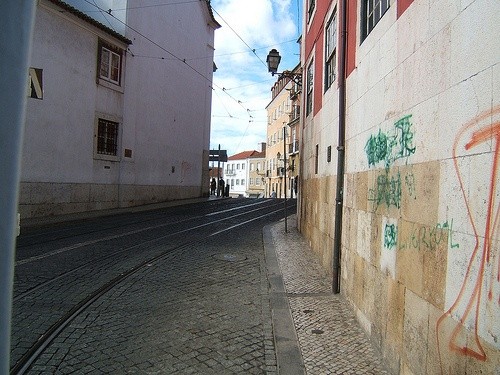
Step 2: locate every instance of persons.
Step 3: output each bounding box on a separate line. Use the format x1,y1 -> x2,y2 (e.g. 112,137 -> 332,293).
219,177 -> 224,197
210,178 -> 216,196
269,190 -> 276,198
